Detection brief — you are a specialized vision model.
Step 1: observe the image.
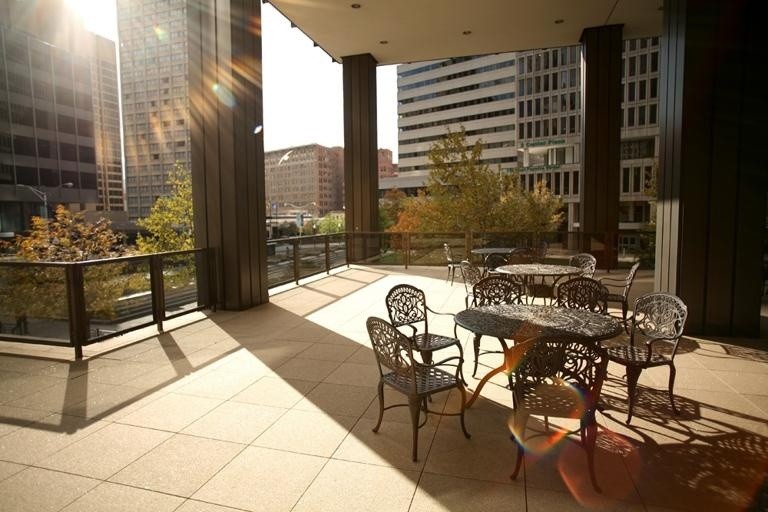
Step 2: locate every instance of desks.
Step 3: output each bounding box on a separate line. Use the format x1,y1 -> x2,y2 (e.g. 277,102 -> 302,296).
454,304 -> 623,412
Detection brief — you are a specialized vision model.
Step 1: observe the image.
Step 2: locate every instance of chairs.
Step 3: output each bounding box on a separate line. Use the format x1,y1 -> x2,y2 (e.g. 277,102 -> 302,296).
504,330 -> 610,493
590,292 -> 690,426
367,317 -> 473,462
557,278 -> 610,380
471,277 -> 521,378
443,242 -> 599,294
385,284 -> 470,388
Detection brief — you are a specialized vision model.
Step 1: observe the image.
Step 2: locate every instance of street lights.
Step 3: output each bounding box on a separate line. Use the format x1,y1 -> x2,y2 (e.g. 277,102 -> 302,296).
16,182 -> 73,219
284,202 -> 316,244
